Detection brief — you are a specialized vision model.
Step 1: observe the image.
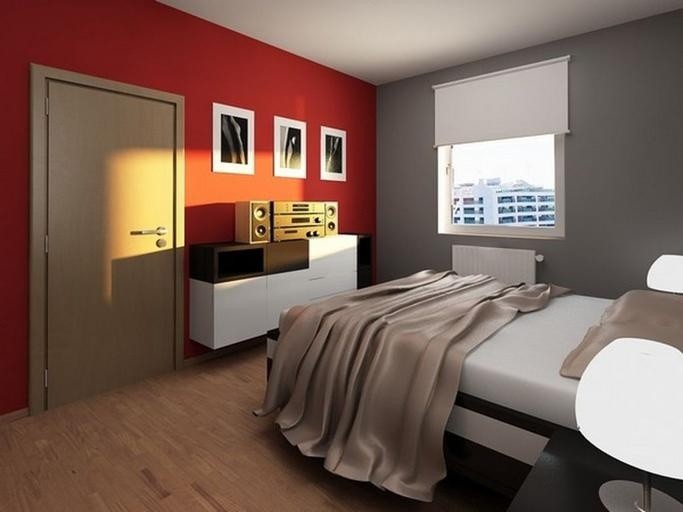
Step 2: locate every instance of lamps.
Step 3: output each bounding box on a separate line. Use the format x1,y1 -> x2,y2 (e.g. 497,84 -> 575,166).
574,337 -> 683,512
646,254 -> 682,293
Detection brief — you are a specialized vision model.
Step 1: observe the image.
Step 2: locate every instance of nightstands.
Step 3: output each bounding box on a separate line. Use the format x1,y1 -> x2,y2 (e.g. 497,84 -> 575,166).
505,426 -> 682,512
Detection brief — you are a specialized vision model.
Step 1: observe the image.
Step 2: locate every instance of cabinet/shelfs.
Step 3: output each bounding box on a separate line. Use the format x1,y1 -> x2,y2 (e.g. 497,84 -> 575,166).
188,233 -> 373,351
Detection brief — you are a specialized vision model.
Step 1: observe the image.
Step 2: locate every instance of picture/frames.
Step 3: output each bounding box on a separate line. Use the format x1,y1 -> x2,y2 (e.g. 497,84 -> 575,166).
212,102 -> 256,176
320,125 -> 347,183
273,115 -> 307,180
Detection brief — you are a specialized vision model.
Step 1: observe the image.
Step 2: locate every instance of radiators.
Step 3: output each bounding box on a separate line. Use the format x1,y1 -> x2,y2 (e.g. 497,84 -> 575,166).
451,245 -> 544,284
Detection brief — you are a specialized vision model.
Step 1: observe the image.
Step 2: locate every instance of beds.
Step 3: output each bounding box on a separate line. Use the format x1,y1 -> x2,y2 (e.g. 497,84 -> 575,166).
265,268 -> 682,512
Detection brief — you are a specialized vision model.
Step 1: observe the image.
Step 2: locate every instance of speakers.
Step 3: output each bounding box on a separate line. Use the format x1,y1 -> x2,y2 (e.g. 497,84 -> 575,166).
325,201 -> 338,236
235,200 -> 272,244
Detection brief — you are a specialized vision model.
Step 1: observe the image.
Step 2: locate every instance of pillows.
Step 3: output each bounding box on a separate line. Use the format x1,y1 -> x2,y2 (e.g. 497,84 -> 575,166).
559,289 -> 682,379
